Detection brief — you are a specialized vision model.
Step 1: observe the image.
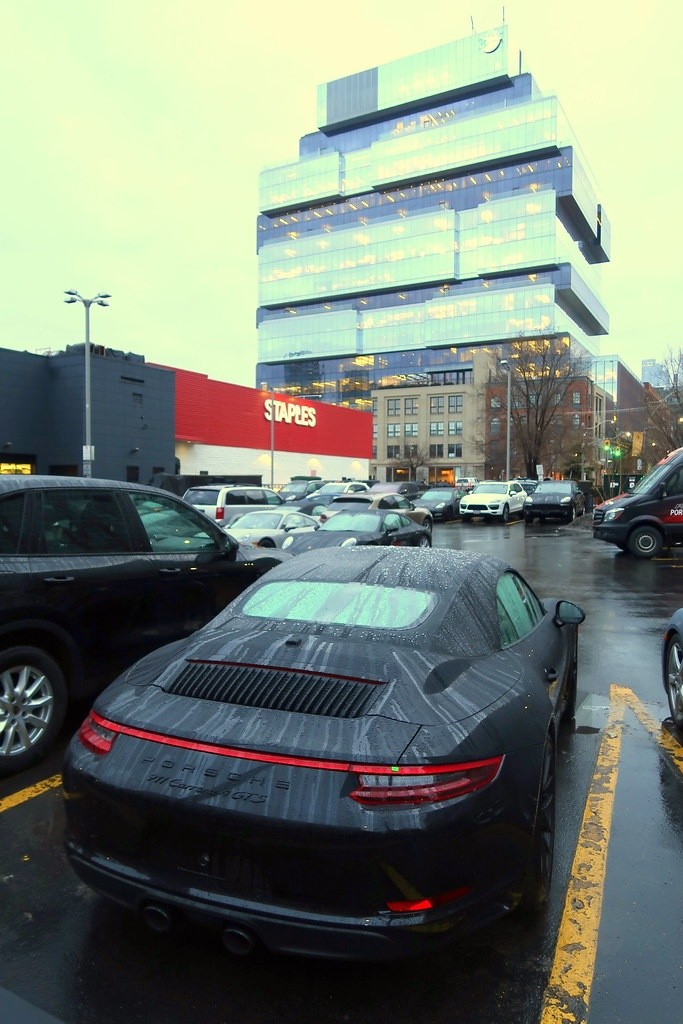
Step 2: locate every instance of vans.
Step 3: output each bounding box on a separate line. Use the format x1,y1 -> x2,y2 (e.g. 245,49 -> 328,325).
592,446 -> 683,558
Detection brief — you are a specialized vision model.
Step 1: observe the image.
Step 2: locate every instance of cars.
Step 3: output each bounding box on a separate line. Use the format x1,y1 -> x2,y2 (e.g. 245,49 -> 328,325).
523,481 -> 586,524
285,508 -> 432,557
432,482 -> 453,488
305,482 -> 370,499
456,476 -> 481,490
283,494 -> 342,516
319,492 -> 432,533
368,482 -> 419,502
195,510 -> 322,548
411,488 -> 466,521
0,475 -> 295,776
661,605 -> 683,735
278,480 -> 325,503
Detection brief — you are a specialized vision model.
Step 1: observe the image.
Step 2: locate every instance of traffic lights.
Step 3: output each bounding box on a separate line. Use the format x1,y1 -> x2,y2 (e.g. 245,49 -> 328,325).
616,447 -> 620,456
604,440 -> 610,450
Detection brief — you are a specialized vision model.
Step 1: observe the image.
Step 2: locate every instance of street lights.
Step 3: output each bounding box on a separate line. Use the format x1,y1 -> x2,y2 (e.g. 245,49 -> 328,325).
501,360 -> 511,482
260,382 -> 275,490
64,288 -> 111,477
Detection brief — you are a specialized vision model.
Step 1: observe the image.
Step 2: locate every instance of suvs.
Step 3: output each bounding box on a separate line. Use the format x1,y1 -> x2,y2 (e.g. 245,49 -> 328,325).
182,483 -> 292,524
459,480 -> 527,522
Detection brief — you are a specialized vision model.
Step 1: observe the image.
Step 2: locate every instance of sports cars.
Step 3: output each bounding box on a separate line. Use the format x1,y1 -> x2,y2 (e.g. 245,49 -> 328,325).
65,547 -> 587,962
